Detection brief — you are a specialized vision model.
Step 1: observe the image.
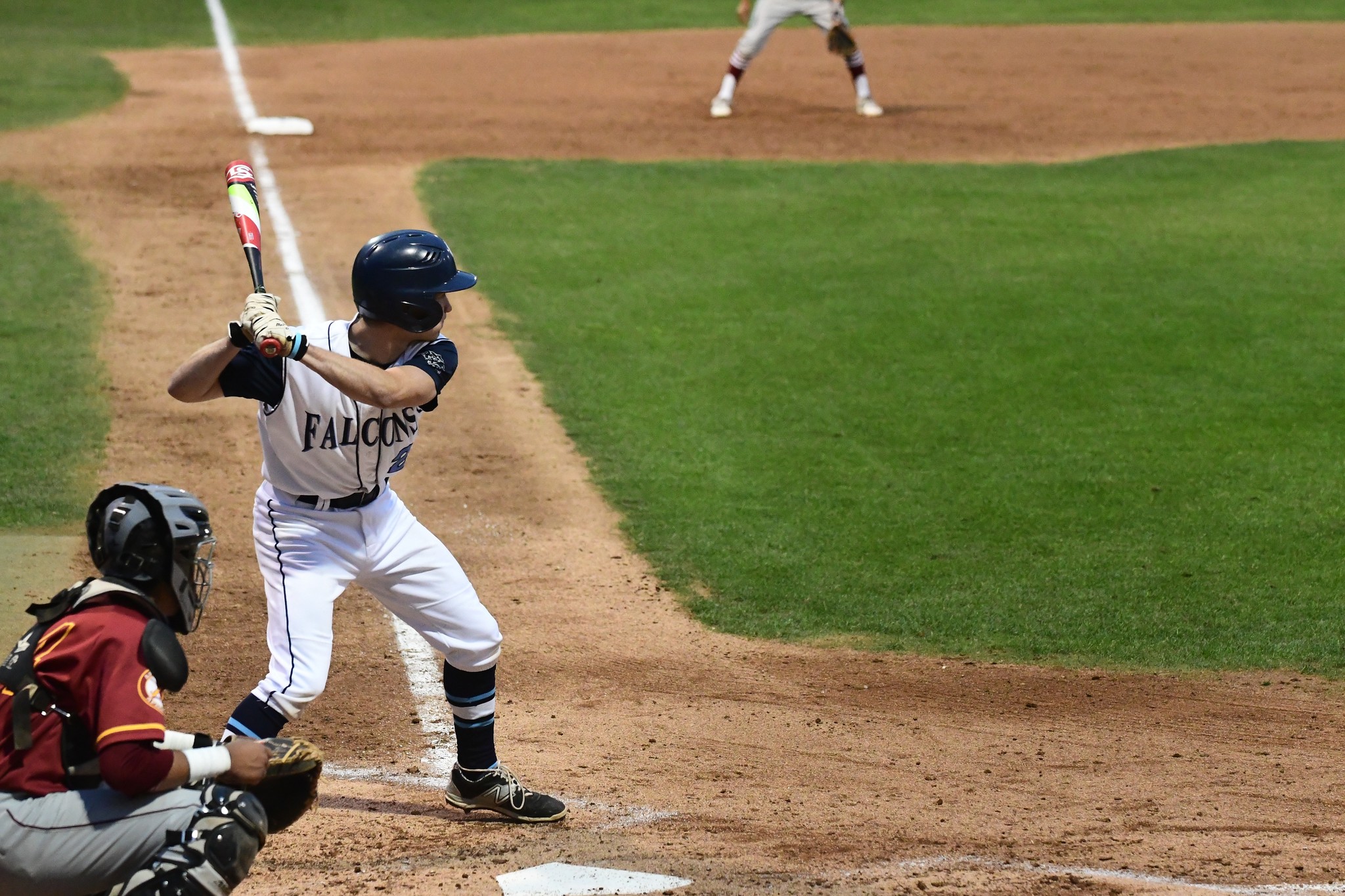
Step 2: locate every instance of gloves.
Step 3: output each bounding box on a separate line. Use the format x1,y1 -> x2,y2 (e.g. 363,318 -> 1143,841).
241,309 -> 309,361
228,293 -> 285,347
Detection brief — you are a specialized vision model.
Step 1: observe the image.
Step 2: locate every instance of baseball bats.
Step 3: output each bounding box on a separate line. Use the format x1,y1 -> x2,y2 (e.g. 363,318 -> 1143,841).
225,160 -> 281,359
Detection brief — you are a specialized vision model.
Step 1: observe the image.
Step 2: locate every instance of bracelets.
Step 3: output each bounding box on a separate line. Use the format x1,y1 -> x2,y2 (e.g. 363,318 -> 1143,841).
180,745 -> 232,783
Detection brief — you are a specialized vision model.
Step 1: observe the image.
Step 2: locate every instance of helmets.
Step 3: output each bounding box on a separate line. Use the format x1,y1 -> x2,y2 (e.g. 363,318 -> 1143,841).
86,482 -> 213,636
351,229 -> 478,333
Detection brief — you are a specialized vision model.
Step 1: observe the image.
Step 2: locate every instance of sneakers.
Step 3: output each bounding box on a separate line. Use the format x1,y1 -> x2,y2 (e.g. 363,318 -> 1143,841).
444,762 -> 567,823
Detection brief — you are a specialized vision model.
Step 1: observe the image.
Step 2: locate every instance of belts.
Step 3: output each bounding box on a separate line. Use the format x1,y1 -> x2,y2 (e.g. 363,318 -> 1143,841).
297,477 -> 390,509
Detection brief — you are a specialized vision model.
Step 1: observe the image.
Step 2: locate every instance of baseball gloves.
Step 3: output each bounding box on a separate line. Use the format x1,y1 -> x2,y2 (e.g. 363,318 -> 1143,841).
238,738 -> 324,835
826,20 -> 859,56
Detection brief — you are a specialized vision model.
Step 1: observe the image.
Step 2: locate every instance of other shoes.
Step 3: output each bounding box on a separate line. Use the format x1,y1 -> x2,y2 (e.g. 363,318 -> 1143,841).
855,99 -> 883,117
710,96 -> 731,118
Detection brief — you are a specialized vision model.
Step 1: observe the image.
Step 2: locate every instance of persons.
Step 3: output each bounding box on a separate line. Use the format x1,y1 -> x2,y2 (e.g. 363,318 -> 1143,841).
710,0 -> 885,118
0,482 -> 272,896
169,228 -> 565,823
